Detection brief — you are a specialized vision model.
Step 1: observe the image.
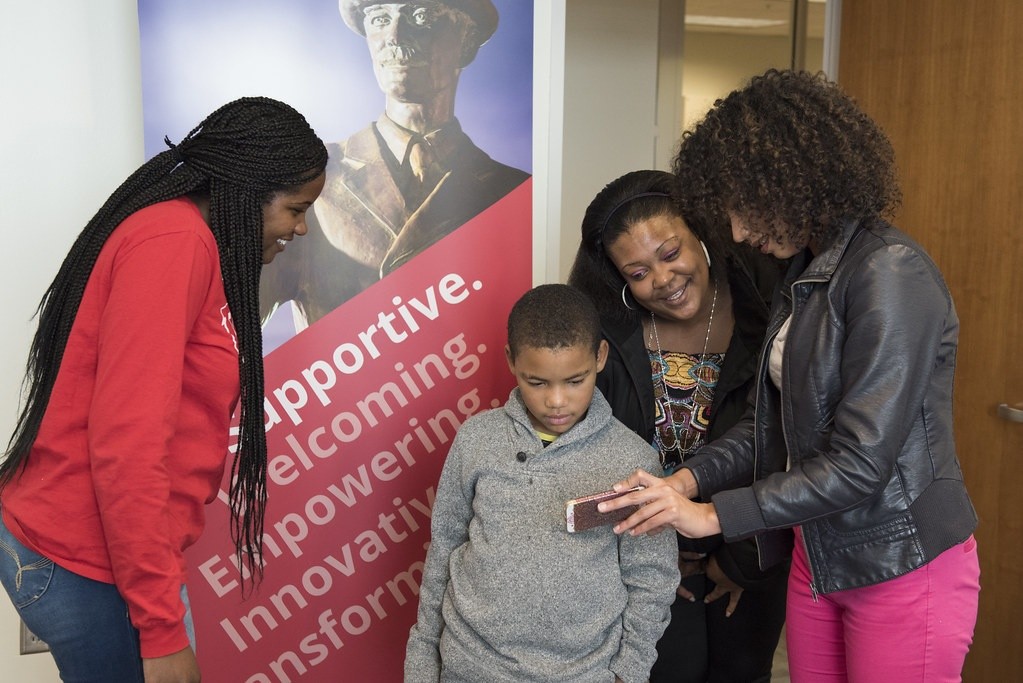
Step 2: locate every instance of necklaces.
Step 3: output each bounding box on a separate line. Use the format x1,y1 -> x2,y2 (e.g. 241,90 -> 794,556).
647,276 -> 719,464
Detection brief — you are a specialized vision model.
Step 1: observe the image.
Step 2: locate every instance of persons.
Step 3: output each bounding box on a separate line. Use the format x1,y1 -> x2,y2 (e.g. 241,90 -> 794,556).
255,0 -> 530,339
565,168 -> 792,683
401,283 -> 681,682
0,95 -> 330,683
596,66 -> 980,683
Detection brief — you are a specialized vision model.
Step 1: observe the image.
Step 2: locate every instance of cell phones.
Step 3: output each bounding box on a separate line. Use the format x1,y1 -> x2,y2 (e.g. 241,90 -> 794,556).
565,487 -> 648,532
